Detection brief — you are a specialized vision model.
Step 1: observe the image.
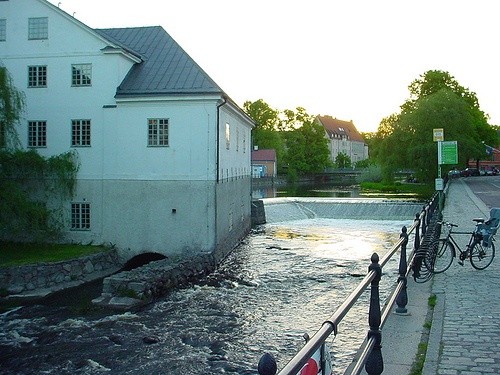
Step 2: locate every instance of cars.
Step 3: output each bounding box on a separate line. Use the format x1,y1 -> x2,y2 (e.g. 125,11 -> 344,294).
478,169 -> 486,176
448,169 -> 463,178
460,170 -> 472,177
485,170 -> 496,176
464,168 -> 480,176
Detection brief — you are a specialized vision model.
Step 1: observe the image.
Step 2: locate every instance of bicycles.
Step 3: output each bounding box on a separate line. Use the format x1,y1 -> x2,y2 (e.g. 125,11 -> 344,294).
424,218 -> 496,274
